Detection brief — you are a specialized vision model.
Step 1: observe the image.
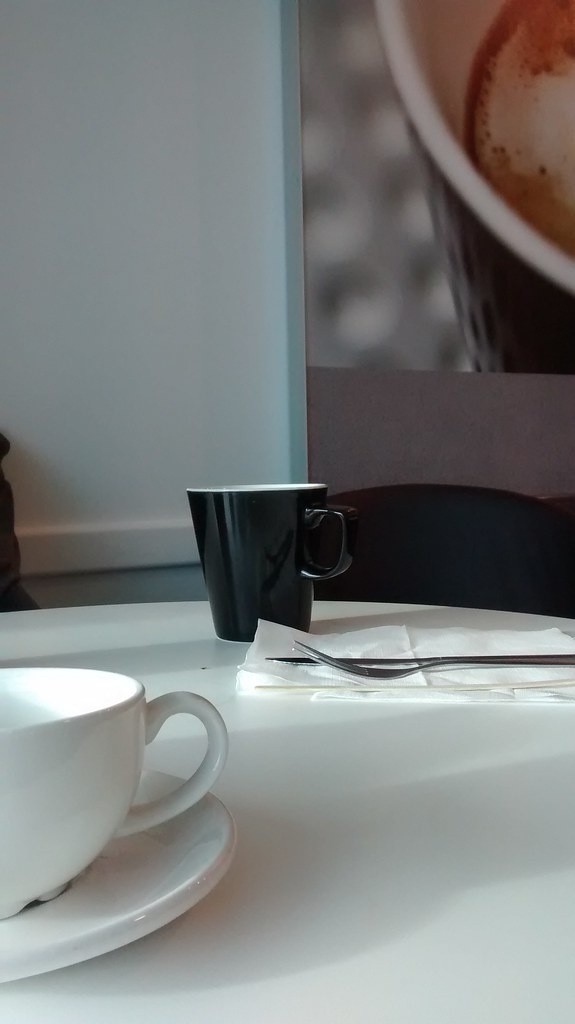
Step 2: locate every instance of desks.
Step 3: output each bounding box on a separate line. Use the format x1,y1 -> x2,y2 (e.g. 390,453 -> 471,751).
0,602 -> 574,1024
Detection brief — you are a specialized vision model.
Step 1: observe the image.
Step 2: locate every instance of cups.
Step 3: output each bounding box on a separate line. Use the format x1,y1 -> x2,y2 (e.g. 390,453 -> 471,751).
369,0 -> 575,376
186,482 -> 359,645
0,666 -> 230,921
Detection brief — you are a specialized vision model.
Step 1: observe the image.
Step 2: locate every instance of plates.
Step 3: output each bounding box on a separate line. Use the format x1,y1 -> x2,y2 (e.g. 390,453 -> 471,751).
0,768 -> 236,985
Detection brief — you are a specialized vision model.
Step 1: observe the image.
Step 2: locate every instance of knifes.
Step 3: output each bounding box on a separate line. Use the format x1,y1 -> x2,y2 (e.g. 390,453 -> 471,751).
265,654 -> 575,666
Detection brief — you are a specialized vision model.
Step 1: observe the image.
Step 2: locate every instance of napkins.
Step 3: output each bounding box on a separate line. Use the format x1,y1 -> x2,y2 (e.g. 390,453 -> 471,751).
235,618 -> 574,703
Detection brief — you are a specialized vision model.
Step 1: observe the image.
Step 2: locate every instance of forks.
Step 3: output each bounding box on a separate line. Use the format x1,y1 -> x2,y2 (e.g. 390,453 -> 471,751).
293,640 -> 575,680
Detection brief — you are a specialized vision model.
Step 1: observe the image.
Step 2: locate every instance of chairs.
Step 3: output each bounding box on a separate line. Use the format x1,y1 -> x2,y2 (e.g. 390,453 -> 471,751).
313,485 -> 575,620
